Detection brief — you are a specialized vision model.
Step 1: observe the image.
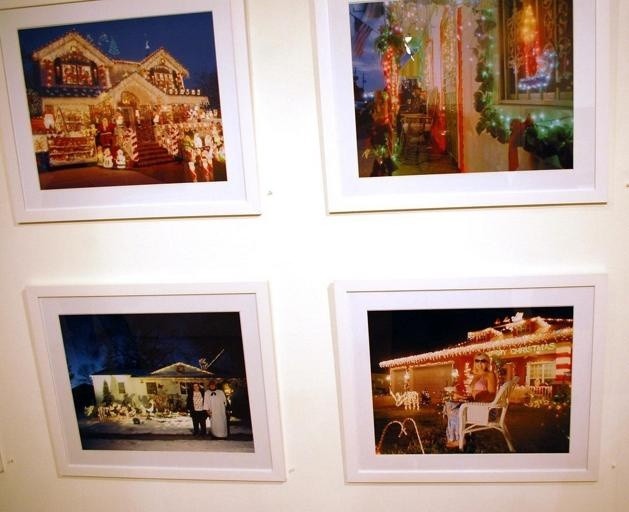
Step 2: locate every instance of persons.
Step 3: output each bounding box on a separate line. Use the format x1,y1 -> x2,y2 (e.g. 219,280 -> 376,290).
204,381 -> 229,439
186,383 -> 208,439
446,351 -> 506,449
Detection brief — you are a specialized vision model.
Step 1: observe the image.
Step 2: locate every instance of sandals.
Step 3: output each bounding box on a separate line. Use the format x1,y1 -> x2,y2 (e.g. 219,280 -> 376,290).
446,439 -> 466,448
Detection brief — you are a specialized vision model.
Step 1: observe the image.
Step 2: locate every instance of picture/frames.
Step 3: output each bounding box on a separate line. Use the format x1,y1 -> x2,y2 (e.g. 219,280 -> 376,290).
21,281 -> 288,484
0,1 -> 266,225
329,274 -> 604,485
311,0 -> 612,217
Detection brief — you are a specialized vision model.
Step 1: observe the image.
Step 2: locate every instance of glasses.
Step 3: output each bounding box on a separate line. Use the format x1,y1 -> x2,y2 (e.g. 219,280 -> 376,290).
474,359 -> 489,363
